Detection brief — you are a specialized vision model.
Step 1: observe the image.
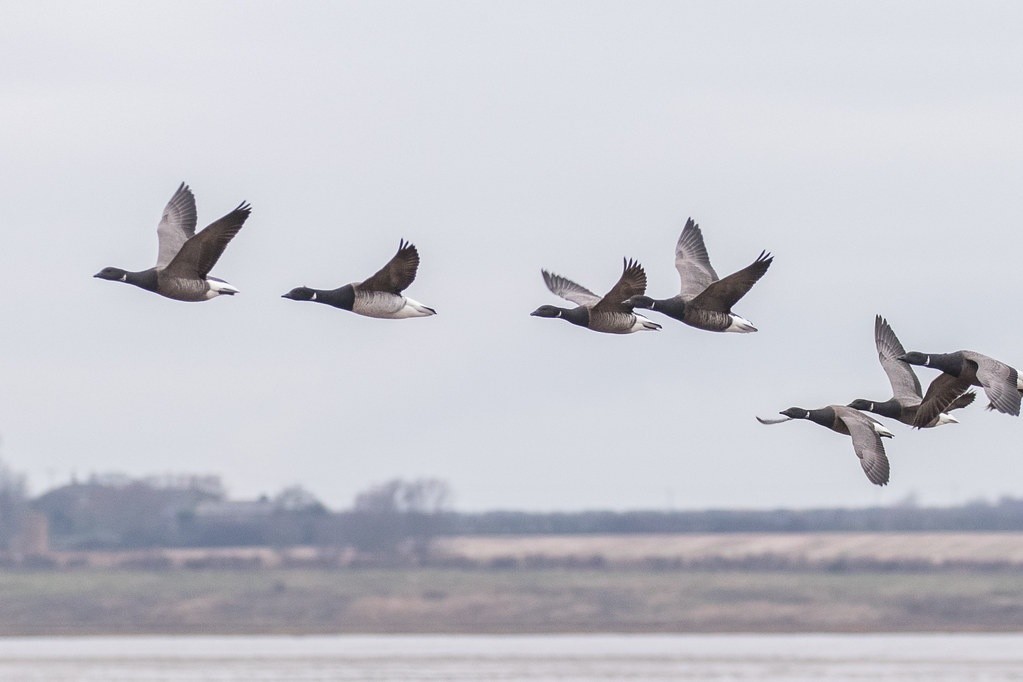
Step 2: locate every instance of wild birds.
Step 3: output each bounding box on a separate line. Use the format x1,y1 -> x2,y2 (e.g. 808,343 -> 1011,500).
281,237 -> 437,319
530,216 -> 774,335
92,181 -> 252,303
756,314 -> 1023,488
